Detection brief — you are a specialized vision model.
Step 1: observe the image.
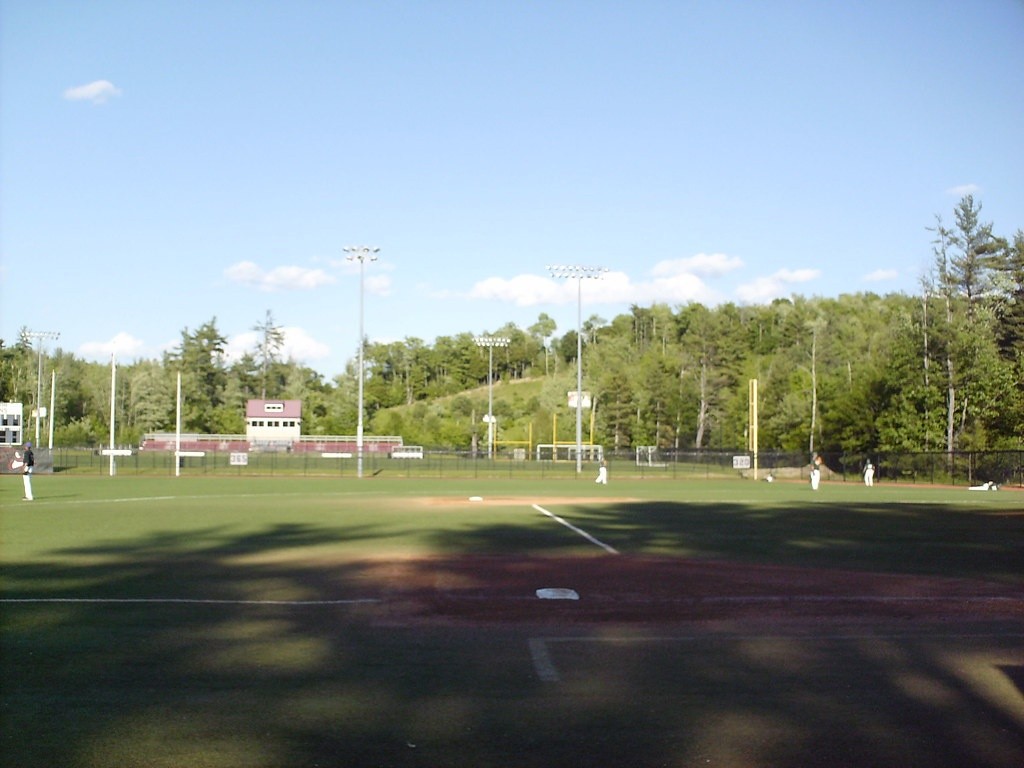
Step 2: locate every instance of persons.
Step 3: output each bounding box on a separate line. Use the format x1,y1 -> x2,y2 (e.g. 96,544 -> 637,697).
595,456 -> 607,485
810,452 -> 821,490
862,459 -> 875,486
22,442 -> 34,501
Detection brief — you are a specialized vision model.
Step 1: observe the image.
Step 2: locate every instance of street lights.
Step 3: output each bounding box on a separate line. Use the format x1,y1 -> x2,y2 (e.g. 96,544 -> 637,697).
544,259 -> 612,473
336,240 -> 383,478
472,337 -> 512,458
16,328 -> 60,448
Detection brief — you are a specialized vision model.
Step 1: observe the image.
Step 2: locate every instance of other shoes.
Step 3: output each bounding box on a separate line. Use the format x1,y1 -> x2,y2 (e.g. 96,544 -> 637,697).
22,497 -> 28,500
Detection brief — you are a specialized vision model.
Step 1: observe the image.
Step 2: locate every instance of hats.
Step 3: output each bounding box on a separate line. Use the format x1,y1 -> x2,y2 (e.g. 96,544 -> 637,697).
24,442 -> 32,447
812,452 -> 817,456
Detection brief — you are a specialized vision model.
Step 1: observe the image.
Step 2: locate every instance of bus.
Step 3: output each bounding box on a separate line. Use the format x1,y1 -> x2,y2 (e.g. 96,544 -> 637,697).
484,441 -> 533,460
535,444 -> 604,463
391,446 -> 424,460
